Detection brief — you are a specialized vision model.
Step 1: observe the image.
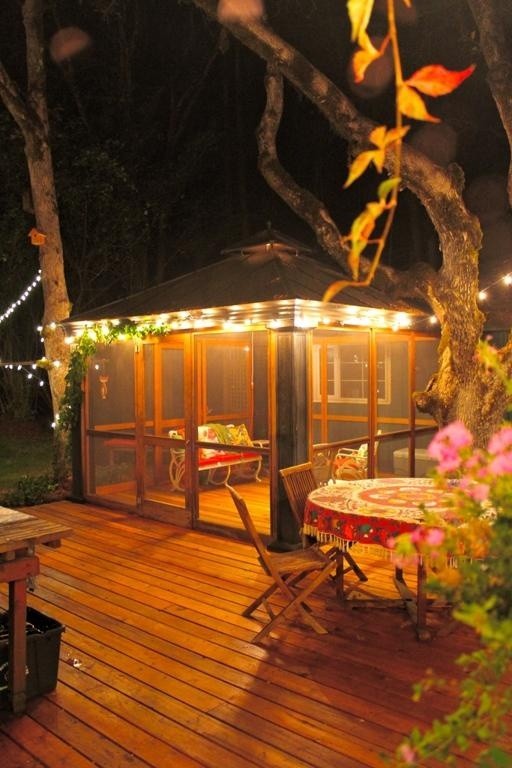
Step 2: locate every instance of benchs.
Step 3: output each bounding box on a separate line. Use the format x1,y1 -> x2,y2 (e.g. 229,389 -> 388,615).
168,430 -> 270,493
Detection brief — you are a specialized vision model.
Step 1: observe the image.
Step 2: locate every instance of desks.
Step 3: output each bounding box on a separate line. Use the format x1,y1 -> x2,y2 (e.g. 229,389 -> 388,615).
300,478 -> 497,643
0,505 -> 73,716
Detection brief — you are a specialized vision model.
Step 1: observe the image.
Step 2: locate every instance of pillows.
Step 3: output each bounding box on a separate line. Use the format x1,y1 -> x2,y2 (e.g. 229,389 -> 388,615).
169,423 -> 253,463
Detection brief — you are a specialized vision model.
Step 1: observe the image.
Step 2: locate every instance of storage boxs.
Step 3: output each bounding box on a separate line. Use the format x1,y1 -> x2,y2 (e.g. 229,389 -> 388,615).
0,602 -> 65,710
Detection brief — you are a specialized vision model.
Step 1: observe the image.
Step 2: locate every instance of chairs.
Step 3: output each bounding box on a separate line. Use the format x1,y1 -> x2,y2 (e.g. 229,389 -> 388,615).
280,462 -> 368,595
331,430 -> 381,483
223,482 -> 337,643
